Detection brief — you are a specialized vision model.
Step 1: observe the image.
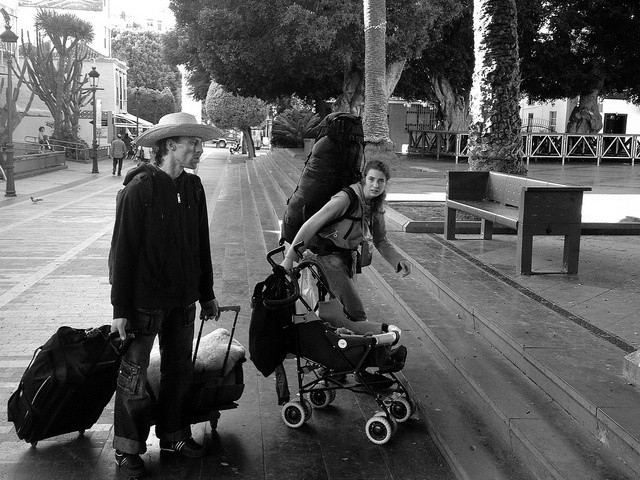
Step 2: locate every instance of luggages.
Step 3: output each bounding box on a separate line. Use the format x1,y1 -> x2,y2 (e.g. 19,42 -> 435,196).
144,305 -> 248,454
7,325 -> 136,446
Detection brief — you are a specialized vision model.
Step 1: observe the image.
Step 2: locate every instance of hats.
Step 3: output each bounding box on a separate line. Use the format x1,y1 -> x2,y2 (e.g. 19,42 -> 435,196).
136,112 -> 224,147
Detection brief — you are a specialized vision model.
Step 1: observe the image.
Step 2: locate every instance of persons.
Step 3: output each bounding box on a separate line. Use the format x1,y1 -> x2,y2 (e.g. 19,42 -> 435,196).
38,126 -> 50,150
282,160 -> 412,388
109,112 -> 220,476
111,133 -> 128,176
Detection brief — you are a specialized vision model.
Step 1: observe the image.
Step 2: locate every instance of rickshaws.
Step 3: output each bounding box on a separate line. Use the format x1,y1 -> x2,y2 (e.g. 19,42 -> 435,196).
156,305 -> 241,439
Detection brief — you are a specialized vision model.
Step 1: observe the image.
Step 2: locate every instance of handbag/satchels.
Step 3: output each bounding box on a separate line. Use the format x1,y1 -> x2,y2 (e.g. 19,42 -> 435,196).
248,266 -> 295,378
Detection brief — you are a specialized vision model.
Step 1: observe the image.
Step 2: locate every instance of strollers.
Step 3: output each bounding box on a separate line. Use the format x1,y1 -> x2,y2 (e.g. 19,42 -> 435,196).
266,240 -> 416,445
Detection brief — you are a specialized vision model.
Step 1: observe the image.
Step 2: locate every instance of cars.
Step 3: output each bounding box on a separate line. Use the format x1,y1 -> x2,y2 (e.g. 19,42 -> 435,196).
212,133 -> 240,148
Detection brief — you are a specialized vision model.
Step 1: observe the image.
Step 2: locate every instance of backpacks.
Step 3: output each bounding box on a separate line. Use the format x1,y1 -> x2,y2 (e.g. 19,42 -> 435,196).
281,112 -> 367,256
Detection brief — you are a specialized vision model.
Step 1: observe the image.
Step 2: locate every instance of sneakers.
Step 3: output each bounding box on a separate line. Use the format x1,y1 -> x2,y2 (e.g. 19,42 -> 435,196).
158,438 -> 212,457
114,450 -> 150,476
317,368 -> 347,380
356,371 -> 393,388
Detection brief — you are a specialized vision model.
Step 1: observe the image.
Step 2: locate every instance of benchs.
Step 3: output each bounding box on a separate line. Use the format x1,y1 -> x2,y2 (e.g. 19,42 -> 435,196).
442,170 -> 590,275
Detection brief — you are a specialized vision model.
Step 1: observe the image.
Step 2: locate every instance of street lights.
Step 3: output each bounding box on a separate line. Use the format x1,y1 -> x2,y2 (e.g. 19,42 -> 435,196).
0,26 -> 19,197
88,67 -> 101,173
